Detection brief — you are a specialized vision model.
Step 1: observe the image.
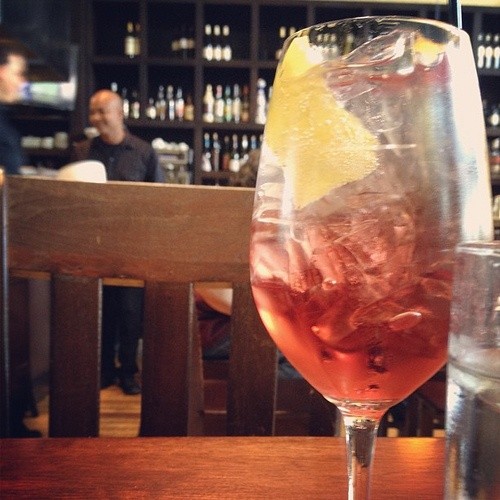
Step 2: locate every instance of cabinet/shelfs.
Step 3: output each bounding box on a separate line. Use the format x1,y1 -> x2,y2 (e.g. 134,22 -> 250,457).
77,0 -> 500,186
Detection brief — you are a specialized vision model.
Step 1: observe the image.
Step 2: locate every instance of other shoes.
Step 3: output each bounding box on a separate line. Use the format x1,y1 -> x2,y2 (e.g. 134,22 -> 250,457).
100,371 -> 115,389
118,368 -> 137,395
10,421 -> 42,437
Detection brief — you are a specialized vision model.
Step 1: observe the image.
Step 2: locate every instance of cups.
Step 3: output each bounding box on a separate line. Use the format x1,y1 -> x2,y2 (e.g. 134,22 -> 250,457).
21,132 -> 69,149
442,239 -> 500,500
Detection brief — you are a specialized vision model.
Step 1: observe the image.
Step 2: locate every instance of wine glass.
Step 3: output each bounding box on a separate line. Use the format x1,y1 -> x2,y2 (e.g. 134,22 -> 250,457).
251,16 -> 496,500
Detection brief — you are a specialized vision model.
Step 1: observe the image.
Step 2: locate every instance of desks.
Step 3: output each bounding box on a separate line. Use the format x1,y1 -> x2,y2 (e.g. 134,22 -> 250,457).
0,434 -> 449,500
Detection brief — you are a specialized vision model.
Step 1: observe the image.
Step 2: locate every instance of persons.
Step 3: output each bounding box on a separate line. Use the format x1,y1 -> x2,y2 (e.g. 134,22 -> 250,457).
80,89 -> 168,397
0,38 -> 43,439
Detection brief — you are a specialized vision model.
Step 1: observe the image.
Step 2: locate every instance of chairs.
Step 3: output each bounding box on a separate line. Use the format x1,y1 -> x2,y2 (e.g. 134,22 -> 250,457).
0,172 -> 337,437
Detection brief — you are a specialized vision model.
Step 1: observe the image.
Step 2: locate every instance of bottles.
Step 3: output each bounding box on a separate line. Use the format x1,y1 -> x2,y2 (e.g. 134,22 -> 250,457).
97,20 -> 297,174
472,30 -> 500,70
